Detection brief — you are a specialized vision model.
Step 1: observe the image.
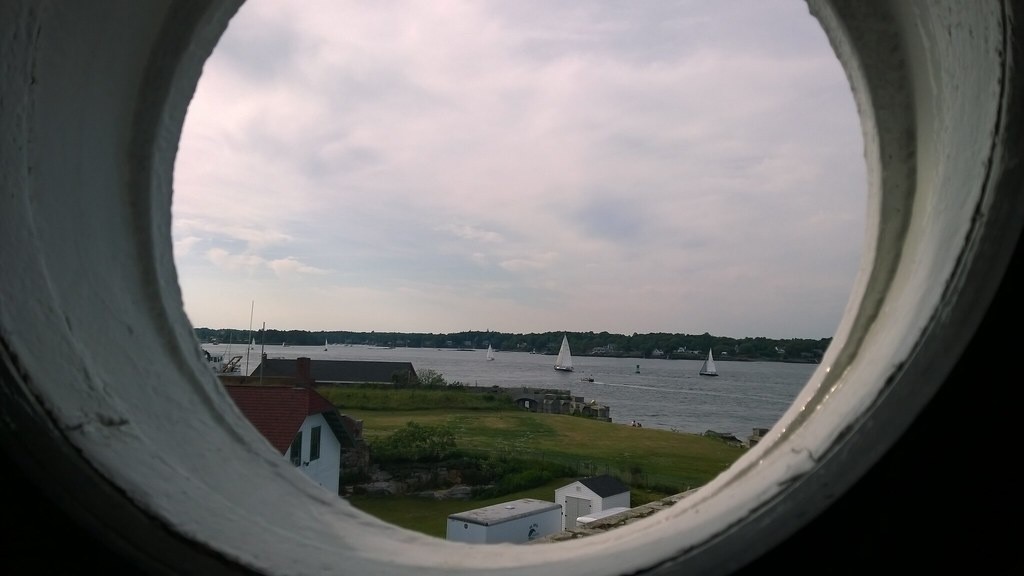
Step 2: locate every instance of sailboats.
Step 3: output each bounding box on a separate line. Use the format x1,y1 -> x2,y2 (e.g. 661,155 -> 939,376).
250,337 -> 256,351
553,332 -> 575,372
699,348 -> 720,376
323,339 -> 328,351
486,343 -> 495,361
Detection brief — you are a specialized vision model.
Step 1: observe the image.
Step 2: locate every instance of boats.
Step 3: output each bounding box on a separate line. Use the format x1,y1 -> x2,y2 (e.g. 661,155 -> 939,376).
202,331 -> 243,376
